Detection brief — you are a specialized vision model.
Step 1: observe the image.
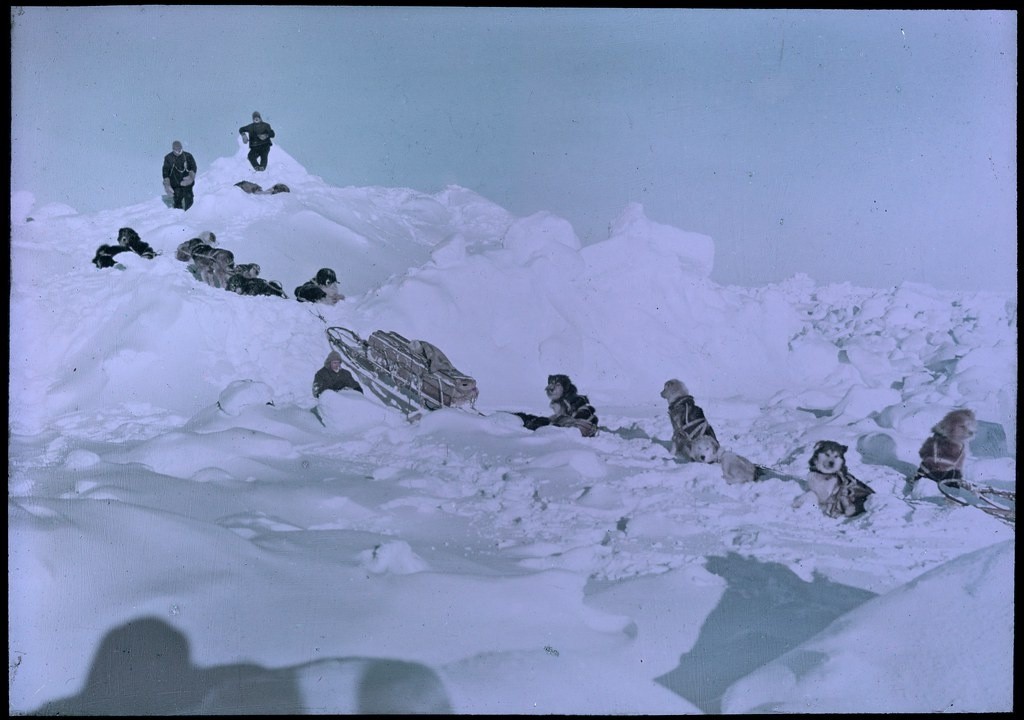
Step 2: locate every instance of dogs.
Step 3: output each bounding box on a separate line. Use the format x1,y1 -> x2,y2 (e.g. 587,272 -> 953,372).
791,440 -> 878,520
508,374 -> 599,438
91,226 -> 346,307
912,408 -> 977,489
233,180 -> 291,195
659,378 -> 769,487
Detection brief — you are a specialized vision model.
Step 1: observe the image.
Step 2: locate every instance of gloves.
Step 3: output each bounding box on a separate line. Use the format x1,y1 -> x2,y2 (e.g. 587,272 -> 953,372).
242,133 -> 248,144
163,178 -> 175,198
258,134 -> 268,140
180,171 -> 195,186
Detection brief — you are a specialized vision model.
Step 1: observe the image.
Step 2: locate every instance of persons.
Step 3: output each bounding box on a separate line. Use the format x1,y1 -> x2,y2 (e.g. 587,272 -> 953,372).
239,111 -> 275,172
312,351 -> 364,399
162,141 -> 197,211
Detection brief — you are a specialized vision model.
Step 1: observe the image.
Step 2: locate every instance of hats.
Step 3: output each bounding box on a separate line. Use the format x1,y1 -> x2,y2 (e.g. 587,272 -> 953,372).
324,351 -> 342,370
252,112 -> 260,119
173,141 -> 182,152
317,268 -> 336,285
